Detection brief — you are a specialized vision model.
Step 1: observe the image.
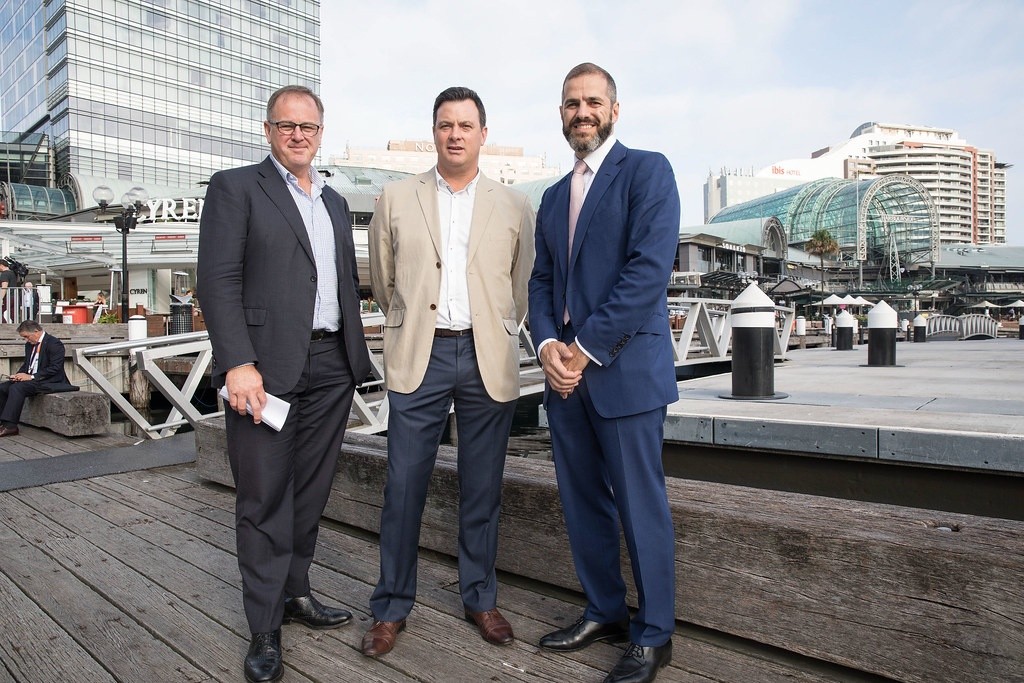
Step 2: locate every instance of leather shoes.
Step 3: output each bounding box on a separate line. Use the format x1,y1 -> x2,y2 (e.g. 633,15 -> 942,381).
465,604 -> 515,645
602,637 -> 672,683
539,615 -> 631,651
243,627 -> 285,683
283,594 -> 353,629
0,425 -> 19,437
361,617 -> 407,655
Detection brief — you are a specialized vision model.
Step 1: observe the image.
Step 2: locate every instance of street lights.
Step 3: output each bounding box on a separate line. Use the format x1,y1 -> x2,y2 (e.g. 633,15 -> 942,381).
93,185 -> 149,322
804,279 -> 821,320
907,284 -> 923,318
737,270 -> 758,288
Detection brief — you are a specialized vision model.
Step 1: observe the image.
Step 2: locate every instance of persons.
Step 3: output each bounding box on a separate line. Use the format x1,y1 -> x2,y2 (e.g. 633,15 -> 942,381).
527,62 -> 680,683
0,259 -> 16,323
186,290 -> 199,317
1008,308 -> 1014,318
197,85 -> 372,683
19,282 -> 39,321
0,320 -> 80,437
93,292 -> 106,319
815,309 -> 820,316
362,86 -> 535,656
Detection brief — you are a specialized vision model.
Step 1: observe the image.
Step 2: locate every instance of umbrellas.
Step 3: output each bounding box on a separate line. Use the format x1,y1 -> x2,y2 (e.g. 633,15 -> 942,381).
813,294 -> 876,315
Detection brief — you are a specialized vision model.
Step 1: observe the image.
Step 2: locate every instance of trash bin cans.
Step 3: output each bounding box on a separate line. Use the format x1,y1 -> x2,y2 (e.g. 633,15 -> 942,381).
128,315 -> 147,354
902,319 -> 909,332
796,316 -> 806,335
170,303 -> 194,334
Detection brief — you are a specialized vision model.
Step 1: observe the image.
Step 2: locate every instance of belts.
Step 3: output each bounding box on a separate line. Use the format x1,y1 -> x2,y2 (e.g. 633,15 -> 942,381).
310,327 -> 344,342
435,328 -> 473,338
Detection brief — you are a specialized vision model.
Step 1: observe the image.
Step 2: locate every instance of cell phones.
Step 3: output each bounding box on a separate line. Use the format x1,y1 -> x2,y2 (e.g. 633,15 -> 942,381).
6,376 -> 16,379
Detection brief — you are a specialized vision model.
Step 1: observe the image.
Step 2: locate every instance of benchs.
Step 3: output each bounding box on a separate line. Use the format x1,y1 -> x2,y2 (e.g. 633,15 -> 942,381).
20,389 -> 110,436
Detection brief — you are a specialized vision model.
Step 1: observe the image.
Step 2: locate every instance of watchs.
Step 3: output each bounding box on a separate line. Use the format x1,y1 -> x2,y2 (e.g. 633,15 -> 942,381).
31,375 -> 35,380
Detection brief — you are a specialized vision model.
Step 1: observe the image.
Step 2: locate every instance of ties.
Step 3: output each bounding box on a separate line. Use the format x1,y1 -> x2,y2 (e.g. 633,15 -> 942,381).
562,159 -> 587,325
29,341 -> 40,375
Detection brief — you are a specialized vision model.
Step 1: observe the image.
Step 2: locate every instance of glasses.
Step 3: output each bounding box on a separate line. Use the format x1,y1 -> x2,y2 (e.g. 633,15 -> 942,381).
267,120 -> 322,137
